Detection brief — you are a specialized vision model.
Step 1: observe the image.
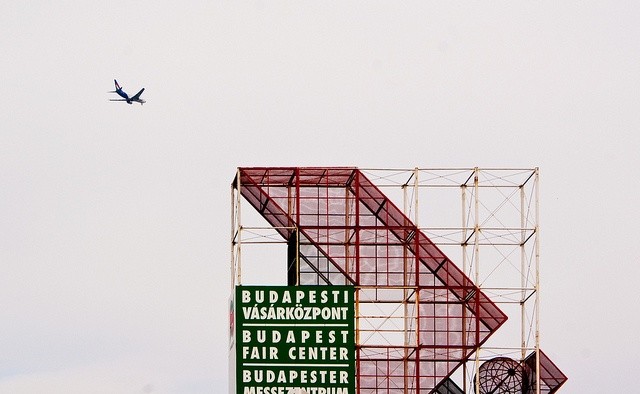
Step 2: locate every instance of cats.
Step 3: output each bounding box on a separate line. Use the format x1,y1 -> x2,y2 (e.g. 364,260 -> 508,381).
107,79 -> 146,105
